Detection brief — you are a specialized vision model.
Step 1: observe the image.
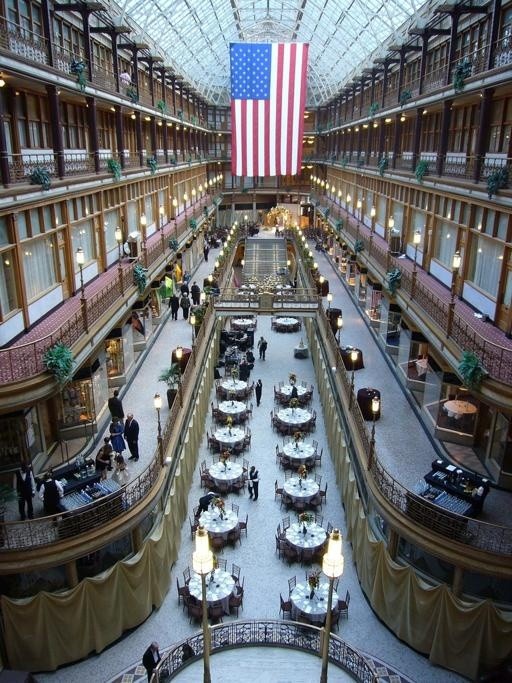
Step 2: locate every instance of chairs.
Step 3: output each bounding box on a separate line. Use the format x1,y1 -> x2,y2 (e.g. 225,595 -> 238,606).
173,313 -> 351,634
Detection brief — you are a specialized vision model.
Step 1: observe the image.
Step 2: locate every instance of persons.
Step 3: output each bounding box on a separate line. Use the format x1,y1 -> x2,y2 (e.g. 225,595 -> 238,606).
203,244 -> 210,262
247,465 -> 261,501
141,640 -> 162,683
238,359 -> 250,382
245,350 -> 256,363
96,388 -> 125,487
158,668 -> 170,683
120,68 -> 131,91
122,411 -> 142,463
166,271 -> 202,322
257,336 -> 268,361
255,378 -> 264,408
36,470 -> 65,513
290,383 -> 298,398
12,459 -> 36,522
194,493 -> 220,518
180,643 -> 195,662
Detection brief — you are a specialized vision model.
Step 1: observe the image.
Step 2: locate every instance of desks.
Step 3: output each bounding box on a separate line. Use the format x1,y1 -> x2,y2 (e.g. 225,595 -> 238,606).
34,456 -> 104,521
423,459 -> 490,514
57,477 -> 125,536
417,486 -> 473,538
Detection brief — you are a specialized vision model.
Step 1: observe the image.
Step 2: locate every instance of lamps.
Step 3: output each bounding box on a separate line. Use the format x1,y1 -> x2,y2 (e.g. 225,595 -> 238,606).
318,526 -> 344,682
192,523 -> 218,683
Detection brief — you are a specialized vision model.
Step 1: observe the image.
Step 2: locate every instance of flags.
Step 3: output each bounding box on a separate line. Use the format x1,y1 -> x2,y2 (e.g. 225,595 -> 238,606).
229,42 -> 310,178
